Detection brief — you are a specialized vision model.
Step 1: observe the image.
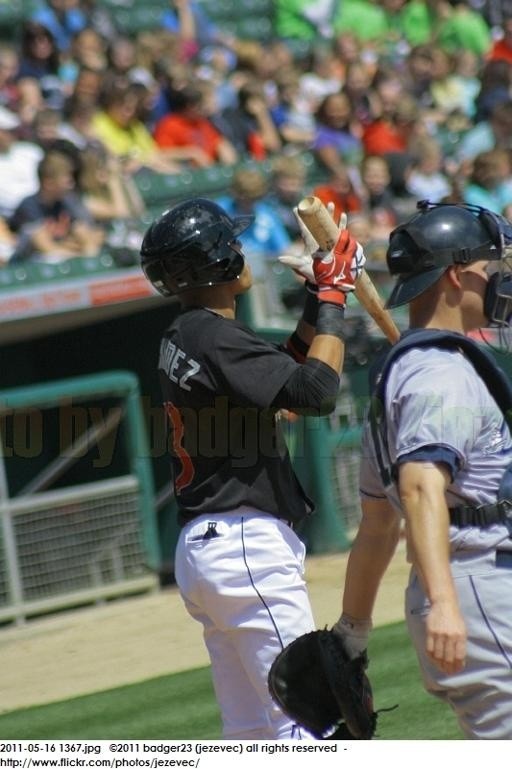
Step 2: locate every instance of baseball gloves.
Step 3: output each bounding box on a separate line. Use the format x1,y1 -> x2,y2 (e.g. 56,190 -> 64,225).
268,630 -> 377,740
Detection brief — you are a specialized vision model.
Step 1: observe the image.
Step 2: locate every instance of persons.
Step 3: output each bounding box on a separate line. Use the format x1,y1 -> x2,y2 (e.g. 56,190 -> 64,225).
0,2 -> 512,263
140,199 -> 512,739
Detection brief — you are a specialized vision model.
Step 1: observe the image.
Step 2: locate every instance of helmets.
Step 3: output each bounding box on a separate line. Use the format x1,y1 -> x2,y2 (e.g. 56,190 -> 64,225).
383,206 -> 493,310
139,199 -> 256,298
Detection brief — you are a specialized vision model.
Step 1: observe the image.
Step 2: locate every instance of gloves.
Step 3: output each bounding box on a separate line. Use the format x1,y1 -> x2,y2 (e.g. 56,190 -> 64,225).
279,202 -> 368,308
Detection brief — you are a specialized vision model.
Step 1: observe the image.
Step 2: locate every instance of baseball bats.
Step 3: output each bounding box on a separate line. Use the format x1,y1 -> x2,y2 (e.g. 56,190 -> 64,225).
298,196 -> 401,346
